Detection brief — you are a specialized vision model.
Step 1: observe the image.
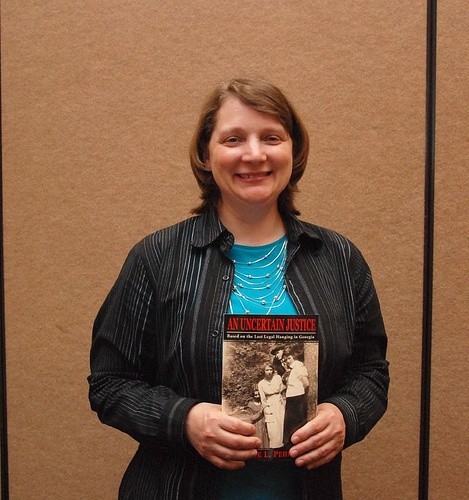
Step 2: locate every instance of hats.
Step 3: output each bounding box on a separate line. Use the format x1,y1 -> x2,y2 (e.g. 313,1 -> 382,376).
270,342 -> 286,355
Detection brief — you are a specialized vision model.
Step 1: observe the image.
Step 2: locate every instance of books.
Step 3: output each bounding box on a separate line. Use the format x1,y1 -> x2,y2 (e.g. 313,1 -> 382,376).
221,312 -> 319,463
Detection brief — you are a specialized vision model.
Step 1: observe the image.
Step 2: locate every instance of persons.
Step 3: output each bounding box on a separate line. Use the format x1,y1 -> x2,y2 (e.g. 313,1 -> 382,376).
248,343 -> 310,450
86,75 -> 392,500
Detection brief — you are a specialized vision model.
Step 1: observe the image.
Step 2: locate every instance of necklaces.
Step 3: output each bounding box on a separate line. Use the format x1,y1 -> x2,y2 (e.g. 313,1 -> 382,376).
227,237 -> 290,315
